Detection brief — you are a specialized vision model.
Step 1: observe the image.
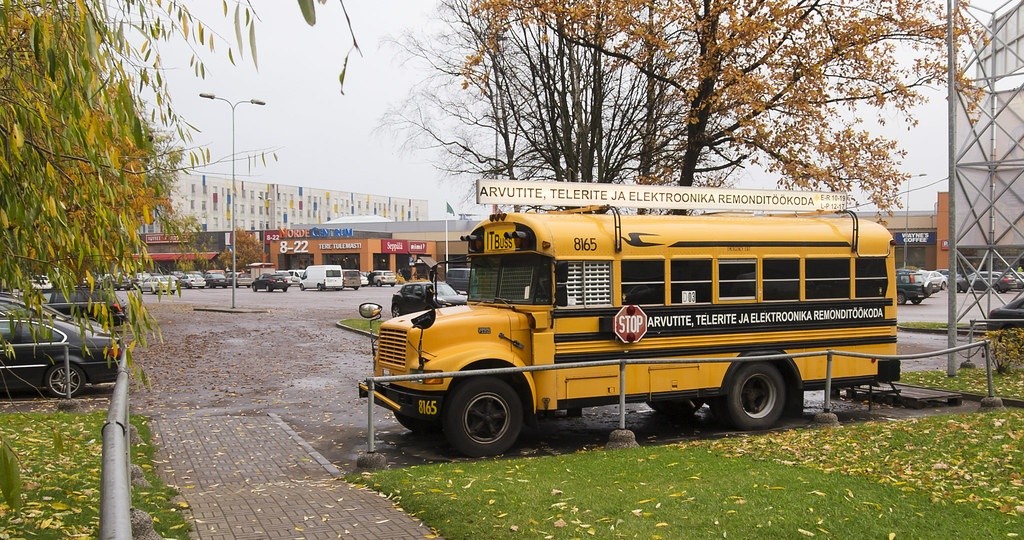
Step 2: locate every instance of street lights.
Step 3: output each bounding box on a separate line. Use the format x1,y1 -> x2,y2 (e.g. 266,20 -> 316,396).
904,173 -> 927,269
200,94 -> 265,308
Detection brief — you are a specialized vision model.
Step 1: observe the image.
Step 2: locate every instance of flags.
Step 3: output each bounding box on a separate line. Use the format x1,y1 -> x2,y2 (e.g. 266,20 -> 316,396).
447,203 -> 455,216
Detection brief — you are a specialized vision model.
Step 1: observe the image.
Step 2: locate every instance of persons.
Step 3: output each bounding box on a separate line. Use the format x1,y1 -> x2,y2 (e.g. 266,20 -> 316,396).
368,271 -> 374,287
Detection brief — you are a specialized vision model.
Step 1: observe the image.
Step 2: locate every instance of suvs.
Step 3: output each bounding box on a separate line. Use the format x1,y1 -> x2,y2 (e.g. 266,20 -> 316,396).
342,269 -> 361,292
896,269 -> 933,305
372,270 -> 397,287
391,282 -> 468,317
276,270 -> 305,286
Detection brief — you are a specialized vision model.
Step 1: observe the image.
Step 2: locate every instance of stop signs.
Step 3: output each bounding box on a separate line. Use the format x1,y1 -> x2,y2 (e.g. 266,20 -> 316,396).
614,304 -> 648,342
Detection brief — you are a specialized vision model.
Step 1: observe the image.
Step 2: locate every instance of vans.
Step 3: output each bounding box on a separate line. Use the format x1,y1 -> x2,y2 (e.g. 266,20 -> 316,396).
446,268 -> 475,294
299,265 -> 343,291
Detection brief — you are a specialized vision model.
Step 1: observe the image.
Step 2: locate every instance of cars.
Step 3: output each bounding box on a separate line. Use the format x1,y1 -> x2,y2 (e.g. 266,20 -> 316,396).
19,269 -> 252,294
252,273 -> 287,292
359,271 -> 369,285
0,284 -> 127,399
917,268 -> 1024,352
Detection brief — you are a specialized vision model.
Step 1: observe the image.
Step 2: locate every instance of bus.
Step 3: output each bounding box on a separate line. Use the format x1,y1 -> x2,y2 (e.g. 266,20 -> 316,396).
359,180 -> 900,459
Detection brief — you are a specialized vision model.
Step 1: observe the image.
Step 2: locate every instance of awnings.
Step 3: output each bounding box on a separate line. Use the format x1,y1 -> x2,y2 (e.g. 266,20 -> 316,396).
133,253 -> 216,260
420,257 -> 437,268
